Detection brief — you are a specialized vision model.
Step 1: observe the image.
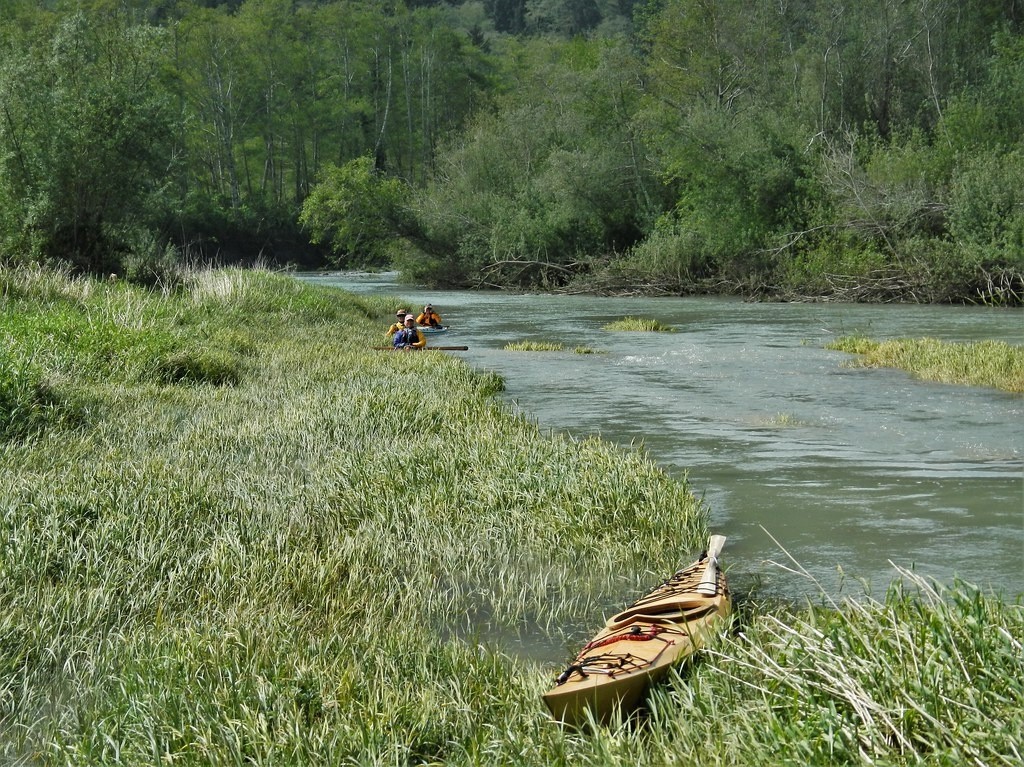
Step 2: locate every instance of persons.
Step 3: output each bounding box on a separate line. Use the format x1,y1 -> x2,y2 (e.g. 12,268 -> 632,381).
386,310 -> 407,337
393,314 -> 426,351
416,303 -> 441,326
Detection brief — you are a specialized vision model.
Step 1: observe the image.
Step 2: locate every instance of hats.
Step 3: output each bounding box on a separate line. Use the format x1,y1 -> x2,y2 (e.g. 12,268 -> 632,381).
397,309 -> 406,315
405,314 -> 414,321
426,303 -> 432,309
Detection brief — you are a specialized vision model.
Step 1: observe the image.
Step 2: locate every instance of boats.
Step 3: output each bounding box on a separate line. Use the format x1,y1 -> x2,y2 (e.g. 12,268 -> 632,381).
395,343 -> 427,351
417,323 -> 448,333
543,536 -> 734,733
